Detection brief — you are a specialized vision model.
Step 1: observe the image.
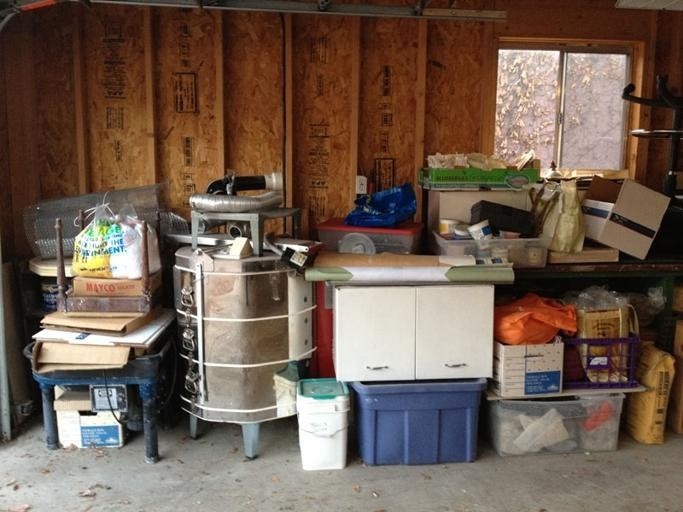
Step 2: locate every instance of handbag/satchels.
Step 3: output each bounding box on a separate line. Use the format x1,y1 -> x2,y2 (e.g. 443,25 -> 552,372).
495,292 -> 577,346
527,177 -> 587,256
343,182 -> 417,230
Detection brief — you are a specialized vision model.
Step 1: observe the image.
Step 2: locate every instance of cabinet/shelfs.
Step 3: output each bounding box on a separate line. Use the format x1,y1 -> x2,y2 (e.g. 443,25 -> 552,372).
330,283 -> 496,381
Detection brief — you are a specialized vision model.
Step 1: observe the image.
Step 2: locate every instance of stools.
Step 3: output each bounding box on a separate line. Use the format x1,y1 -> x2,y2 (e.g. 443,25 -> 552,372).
54,209 -> 163,315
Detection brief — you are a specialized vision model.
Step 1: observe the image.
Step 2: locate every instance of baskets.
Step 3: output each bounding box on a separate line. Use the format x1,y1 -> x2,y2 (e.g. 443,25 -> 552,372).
563,332 -> 639,389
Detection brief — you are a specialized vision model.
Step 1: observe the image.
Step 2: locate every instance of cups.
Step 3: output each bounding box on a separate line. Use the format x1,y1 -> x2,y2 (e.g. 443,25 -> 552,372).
468,218 -> 496,250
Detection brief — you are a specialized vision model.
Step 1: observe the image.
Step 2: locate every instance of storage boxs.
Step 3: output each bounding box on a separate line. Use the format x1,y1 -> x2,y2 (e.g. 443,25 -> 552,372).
53,386 -> 129,449
579,174 -> 673,261
347,335 -> 627,468
317,188 -> 550,270
